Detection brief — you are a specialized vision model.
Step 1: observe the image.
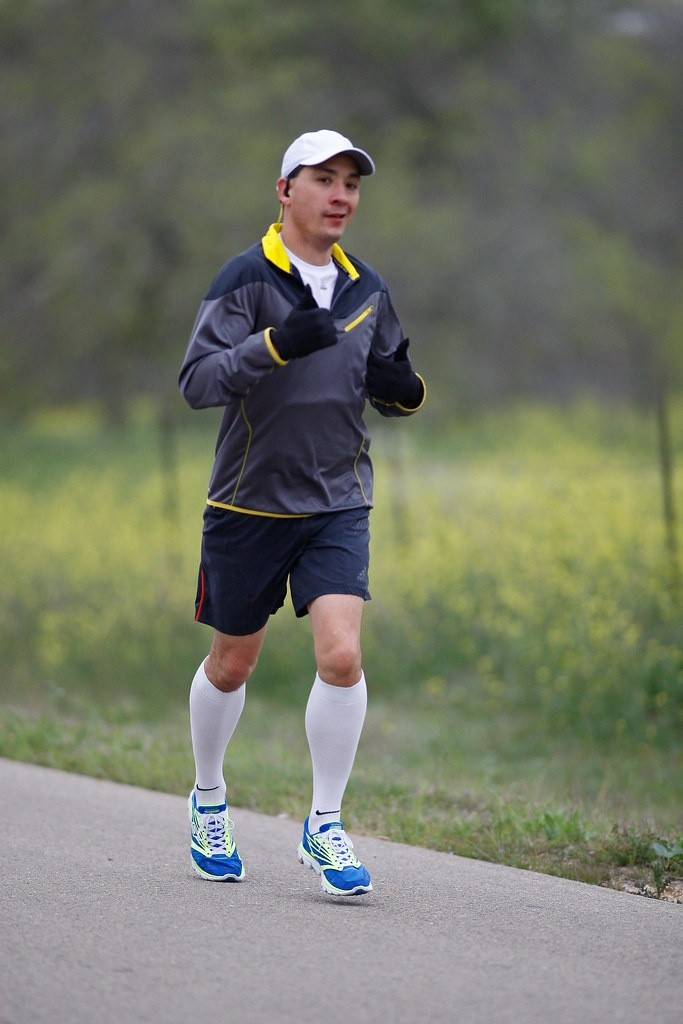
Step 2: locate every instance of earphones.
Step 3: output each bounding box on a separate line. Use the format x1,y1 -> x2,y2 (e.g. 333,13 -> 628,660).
283,182 -> 290,198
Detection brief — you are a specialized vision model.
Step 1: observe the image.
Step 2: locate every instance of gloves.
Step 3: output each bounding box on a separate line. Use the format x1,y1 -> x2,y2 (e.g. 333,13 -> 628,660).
365,338 -> 424,409
271,284 -> 337,360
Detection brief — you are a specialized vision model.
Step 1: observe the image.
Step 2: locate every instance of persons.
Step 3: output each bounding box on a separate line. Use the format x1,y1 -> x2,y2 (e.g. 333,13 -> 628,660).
180,130 -> 426,895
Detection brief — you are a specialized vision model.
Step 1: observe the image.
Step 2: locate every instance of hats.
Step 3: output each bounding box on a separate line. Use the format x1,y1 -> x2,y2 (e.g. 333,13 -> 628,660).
280,130 -> 375,179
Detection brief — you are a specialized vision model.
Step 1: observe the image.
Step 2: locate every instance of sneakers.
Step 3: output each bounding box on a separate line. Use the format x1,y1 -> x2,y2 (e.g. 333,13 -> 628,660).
188,789 -> 245,882
297,816 -> 373,896
